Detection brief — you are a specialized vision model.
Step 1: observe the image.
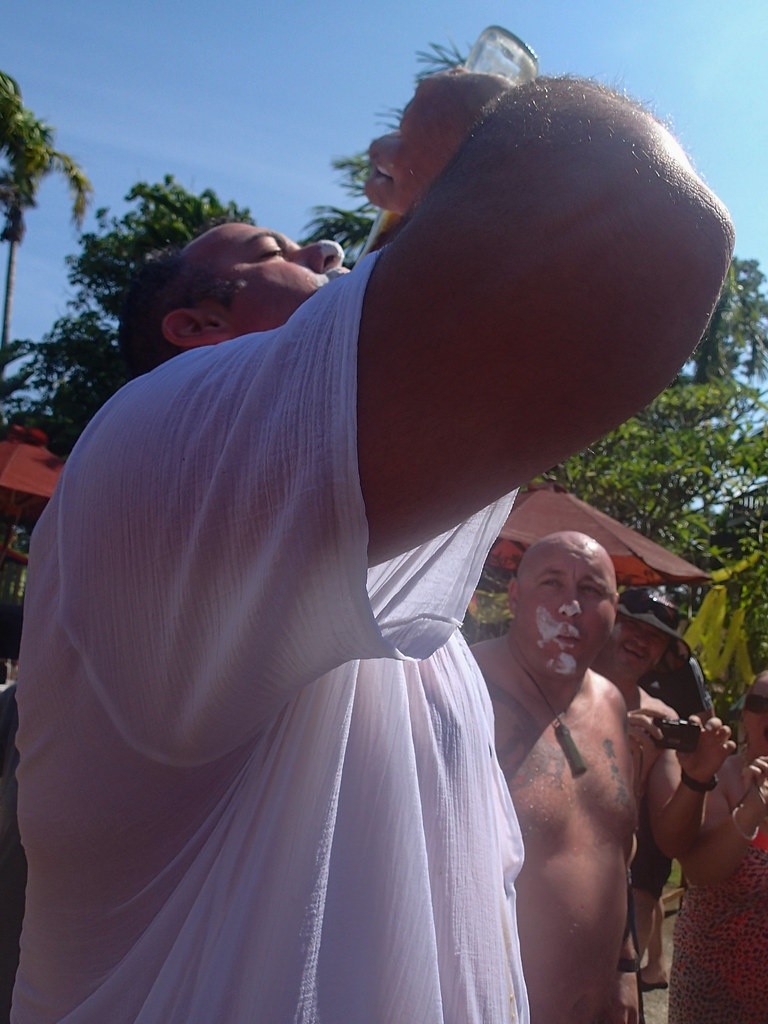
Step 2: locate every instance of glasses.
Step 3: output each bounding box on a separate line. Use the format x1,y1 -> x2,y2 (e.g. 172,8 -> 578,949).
618,586 -> 679,631
743,693 -> 768,714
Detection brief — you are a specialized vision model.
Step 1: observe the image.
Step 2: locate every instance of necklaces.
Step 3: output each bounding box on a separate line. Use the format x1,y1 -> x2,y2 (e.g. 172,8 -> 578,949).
509,645 -> 586,778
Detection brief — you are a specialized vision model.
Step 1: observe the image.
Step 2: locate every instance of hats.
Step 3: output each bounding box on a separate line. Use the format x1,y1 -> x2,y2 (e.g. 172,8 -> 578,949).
617,585 -> 691,677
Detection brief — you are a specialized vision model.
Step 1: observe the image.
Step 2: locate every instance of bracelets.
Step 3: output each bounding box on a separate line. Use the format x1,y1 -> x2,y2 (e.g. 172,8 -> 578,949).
732,777 -> 757,814
681,768 -> 719,793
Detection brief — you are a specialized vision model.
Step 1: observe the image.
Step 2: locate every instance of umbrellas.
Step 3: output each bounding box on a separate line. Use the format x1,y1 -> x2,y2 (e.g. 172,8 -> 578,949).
0,420 -> 65,524
482,484 -> 713,587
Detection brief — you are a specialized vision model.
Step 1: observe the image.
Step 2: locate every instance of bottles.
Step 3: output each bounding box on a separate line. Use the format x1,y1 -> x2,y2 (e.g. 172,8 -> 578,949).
356,25 -> 539,262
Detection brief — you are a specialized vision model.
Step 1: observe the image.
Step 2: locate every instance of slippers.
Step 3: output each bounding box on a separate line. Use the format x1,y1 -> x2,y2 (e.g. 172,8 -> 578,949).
640,978 -> 669,992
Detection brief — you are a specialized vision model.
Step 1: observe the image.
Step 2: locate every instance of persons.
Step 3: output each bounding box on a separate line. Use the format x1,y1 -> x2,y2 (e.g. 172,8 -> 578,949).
467,533 -> 767,1024
7,73 -> 736,1024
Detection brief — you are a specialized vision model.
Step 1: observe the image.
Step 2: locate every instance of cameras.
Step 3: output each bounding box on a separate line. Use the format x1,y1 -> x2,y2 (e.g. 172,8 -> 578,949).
650,719 -> 702,754
